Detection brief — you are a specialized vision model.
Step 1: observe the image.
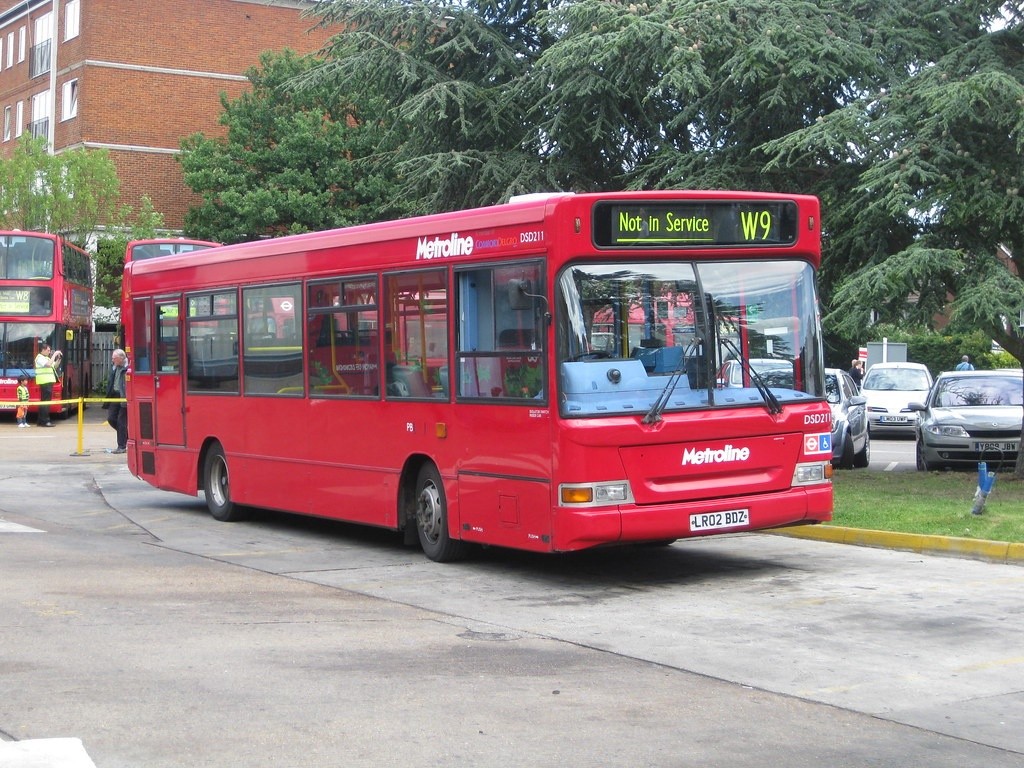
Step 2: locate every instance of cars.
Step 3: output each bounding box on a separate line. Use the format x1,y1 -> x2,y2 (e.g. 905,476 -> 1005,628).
910,369 -> 1024,474
715,358 -> 796,389
750,366 -> 871,474
858,361 -> 935,443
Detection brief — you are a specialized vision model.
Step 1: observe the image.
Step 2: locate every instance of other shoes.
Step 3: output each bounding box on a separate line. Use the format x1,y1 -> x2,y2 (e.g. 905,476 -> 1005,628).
37,422 -> 56,427
18,423 -> 30,428
111,447 -> 127,454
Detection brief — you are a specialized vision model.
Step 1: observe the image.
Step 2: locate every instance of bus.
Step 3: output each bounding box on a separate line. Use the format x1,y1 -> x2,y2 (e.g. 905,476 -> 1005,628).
0,229 -> 92,420
589,286 -> 749,366
221,286 -> 456,394
121,234 -> 241,337
119,185 -> 841,561
465,259 -> 547,403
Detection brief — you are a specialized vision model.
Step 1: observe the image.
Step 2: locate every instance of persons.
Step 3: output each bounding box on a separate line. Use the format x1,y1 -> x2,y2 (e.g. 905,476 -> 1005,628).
955,354 -> 976,371
848,359 -> 867,393
14,374 -> 32,429
99,348 -> 131,455
33,342 -> 63,427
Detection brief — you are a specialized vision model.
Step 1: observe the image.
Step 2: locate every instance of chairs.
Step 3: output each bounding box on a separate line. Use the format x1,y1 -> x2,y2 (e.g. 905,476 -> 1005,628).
148,325 -> 540,401
